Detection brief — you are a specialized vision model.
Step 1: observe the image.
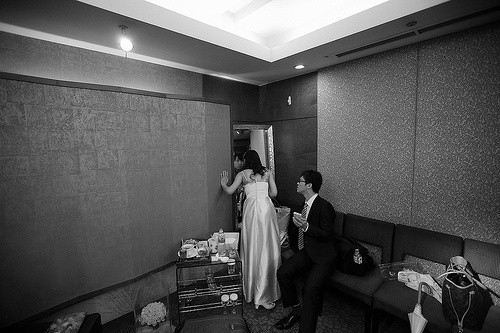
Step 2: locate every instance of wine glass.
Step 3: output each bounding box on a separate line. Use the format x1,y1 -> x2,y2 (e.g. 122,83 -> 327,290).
198,246 -> 208,260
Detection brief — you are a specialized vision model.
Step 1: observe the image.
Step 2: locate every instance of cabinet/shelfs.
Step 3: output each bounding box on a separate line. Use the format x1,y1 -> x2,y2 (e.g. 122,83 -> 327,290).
177,250 -> 244,323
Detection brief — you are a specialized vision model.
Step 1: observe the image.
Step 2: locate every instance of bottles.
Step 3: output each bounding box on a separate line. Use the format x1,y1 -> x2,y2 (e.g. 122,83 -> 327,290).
204,266 -> 217,288
219,229 -> 225,252
210,238 -> 219,262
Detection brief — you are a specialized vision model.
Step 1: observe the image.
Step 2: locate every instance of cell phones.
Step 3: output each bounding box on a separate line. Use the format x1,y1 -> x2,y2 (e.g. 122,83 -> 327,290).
293,212 -> 302,222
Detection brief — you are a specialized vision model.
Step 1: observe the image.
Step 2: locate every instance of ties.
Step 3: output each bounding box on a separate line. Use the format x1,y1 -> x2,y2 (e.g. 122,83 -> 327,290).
298,203 -> 308,250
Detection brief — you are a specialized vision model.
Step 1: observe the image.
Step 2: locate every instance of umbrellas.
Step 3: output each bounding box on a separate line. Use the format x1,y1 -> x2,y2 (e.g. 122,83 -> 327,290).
408,282 -> 431,333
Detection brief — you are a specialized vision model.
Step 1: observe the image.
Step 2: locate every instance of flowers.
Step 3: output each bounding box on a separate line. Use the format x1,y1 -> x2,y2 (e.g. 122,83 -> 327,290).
46,311 -> 88,333
138,301 -> 169,326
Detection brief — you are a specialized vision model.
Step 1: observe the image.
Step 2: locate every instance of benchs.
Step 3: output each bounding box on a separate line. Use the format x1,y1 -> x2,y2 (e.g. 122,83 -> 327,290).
331,211 -> 500,333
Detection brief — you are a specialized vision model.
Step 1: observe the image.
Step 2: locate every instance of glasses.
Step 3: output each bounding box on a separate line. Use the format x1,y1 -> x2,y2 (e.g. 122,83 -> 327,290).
298,179 -> 306,183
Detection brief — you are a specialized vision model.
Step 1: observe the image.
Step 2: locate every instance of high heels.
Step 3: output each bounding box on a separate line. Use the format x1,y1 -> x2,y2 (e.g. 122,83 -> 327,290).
254,299 -> 275,309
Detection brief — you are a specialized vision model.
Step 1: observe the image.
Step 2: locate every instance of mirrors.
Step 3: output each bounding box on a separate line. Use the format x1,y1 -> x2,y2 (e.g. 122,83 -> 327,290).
234,123 -> 275,223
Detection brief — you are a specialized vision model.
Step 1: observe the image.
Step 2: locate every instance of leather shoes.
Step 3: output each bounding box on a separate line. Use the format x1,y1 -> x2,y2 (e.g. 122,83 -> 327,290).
275,312 -> 302,330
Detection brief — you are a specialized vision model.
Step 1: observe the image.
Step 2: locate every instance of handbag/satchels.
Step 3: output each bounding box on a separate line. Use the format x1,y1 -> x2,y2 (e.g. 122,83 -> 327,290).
437,256 -> 500,333
270,197 -> 291,241
334,248 -> 377,277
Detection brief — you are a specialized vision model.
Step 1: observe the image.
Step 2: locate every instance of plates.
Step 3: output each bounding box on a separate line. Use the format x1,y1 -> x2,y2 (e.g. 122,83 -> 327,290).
182,239 -> 198,246
178,248 -> 198,258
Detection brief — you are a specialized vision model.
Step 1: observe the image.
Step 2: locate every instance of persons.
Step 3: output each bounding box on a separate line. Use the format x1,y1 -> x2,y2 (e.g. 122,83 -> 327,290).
220,150 -> 281,309
233,153 -> 244,182
274,170 -> 335,333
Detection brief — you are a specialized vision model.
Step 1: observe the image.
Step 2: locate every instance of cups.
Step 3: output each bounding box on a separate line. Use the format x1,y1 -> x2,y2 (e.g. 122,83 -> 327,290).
179,249 -> 188,262
229,243 -> 237,258
227,262 -> 236,274
219,244 -> 228,257
180,243 -> 195,256
